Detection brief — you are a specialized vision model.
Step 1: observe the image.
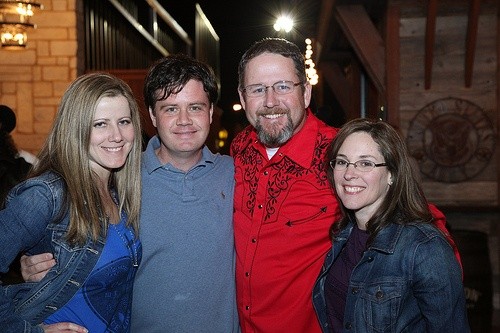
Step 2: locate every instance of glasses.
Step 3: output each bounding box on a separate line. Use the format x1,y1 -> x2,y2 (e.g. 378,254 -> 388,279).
240,80 -> 303,98
330,159 -> 389,171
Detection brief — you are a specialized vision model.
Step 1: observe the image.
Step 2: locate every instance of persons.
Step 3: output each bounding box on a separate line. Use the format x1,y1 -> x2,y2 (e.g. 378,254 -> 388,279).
230,37 -> 464,333
310,118 -> 464,333
0,53 -> 241,333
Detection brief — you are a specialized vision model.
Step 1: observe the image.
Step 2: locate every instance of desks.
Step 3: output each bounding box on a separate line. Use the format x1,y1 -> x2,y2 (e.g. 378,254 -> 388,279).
412,179 -> 499,333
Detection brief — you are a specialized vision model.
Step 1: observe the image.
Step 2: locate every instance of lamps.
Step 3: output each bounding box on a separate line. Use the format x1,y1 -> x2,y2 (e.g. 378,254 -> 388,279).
0,0 -> 44,54
